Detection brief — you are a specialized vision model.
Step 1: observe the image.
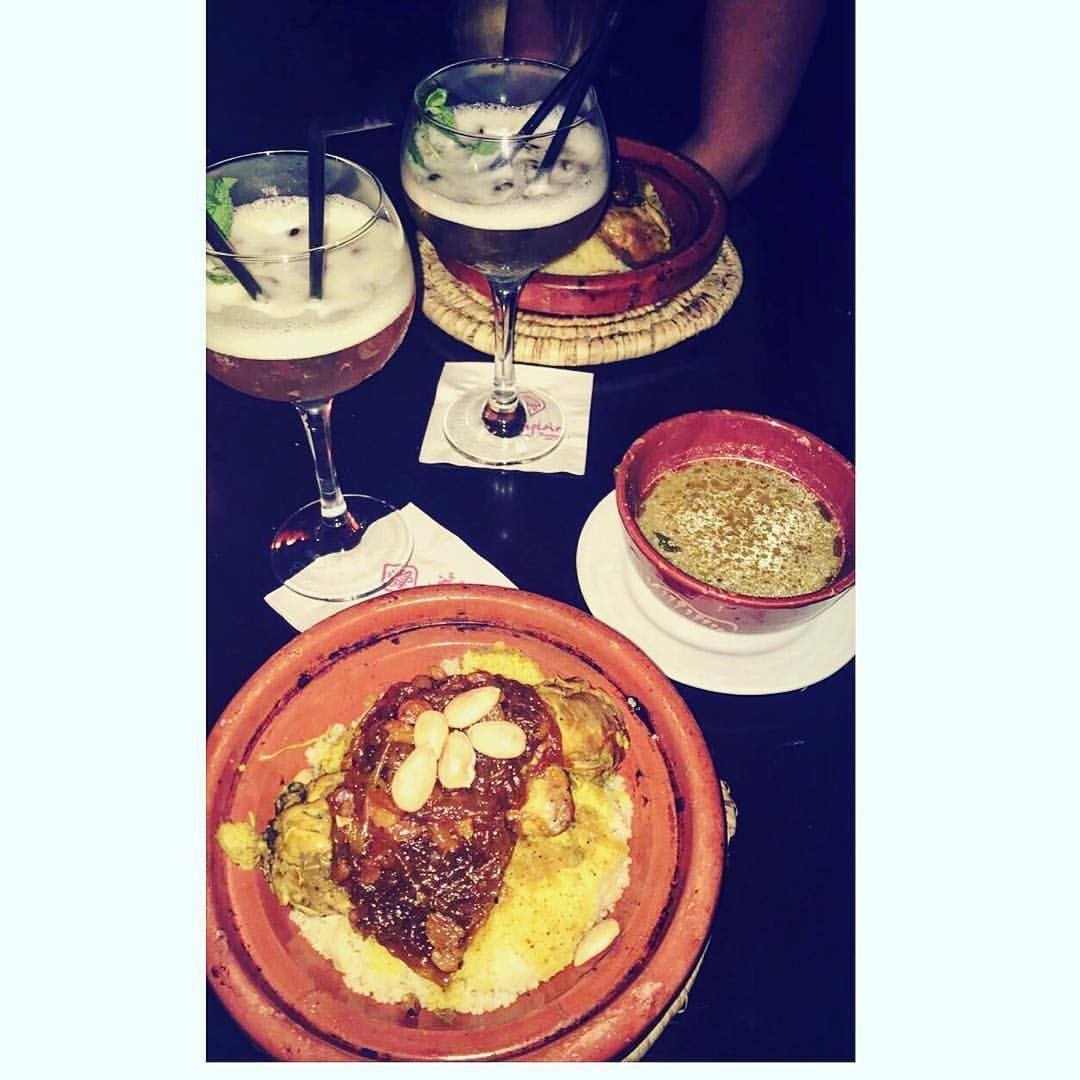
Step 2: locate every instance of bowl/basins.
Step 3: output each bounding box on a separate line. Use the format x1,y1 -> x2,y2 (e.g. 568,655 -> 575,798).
438,136 -> 726,315
615,408 -> 855,633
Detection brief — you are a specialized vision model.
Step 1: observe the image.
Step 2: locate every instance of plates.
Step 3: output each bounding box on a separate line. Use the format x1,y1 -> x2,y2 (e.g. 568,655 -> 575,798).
206,585 -> 727,1063
576,489 -> 857,696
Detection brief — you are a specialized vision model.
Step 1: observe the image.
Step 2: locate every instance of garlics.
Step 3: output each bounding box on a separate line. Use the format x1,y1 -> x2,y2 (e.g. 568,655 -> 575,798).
392,686 -> 526,813
574,919 -> 620,968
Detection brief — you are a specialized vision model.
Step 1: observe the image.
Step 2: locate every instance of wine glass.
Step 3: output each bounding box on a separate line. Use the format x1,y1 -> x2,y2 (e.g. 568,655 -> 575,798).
398,56 -> 615,466
206,149 -> 415,601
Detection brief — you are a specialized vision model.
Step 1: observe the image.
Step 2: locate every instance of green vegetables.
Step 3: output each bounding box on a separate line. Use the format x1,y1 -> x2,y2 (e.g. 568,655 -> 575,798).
408,88 -> 501,171
206,175 -> 241,284
